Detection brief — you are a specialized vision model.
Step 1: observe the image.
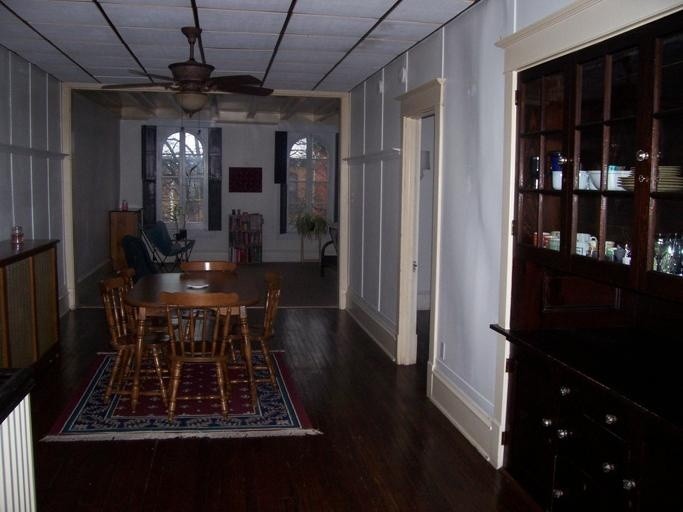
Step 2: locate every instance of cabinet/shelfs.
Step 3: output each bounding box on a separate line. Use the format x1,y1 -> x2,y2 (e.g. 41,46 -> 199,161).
0,239 -> 61,370
228,214 -> 264,265
490,323 -> 683,512
511,9 -> 683,307
110,208 -> 144,267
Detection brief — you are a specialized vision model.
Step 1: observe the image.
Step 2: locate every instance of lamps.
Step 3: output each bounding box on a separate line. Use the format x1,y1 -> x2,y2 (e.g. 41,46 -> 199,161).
174,90 -> 210,113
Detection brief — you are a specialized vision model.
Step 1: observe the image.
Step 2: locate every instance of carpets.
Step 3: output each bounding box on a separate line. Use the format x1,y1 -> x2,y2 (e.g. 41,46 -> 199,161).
40,340 -> 323,441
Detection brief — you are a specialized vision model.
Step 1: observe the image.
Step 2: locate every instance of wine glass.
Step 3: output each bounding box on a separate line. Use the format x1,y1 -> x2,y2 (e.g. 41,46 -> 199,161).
653,232 -> 683,275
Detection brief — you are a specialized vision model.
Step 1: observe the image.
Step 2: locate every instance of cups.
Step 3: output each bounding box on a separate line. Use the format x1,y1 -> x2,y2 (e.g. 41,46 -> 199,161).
533,230 -> 616,258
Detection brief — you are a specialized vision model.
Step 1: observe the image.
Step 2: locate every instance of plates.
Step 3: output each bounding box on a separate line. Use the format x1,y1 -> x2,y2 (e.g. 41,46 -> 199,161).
617,163 -> 682,192
186,283 -> 209,289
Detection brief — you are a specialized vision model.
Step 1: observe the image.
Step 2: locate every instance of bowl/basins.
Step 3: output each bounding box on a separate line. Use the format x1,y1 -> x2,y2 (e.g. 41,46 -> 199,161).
550,170 -> 630,192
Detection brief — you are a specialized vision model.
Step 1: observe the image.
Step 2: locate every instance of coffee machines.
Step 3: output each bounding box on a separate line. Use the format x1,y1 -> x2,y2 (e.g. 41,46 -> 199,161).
525,155 -> 539,189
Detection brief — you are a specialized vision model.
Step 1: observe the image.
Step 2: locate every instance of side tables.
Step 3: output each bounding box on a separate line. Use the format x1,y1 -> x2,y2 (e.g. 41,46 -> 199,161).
172,238 -> 195,262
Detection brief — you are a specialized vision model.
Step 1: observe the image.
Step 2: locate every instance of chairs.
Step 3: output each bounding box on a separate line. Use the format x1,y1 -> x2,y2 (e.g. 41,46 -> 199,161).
321,226 -> 339,277
121,235 -> 153,282
142,220 -> 195,274
99,261 -> 280,421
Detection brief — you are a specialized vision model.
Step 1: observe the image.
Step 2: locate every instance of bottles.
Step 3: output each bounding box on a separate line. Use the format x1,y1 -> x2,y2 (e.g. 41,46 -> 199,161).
10,225 -> 23,244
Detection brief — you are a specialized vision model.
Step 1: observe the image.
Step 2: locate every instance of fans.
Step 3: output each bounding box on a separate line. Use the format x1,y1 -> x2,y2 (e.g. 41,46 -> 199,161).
101,26 -> 274,97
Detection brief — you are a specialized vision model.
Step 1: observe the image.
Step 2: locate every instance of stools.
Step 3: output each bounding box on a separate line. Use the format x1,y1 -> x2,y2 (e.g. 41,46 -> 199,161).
300,230 -> 325,264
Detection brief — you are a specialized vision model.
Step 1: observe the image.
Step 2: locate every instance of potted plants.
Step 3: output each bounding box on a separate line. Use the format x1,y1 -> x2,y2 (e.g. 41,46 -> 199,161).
298,212 -> 327,233
